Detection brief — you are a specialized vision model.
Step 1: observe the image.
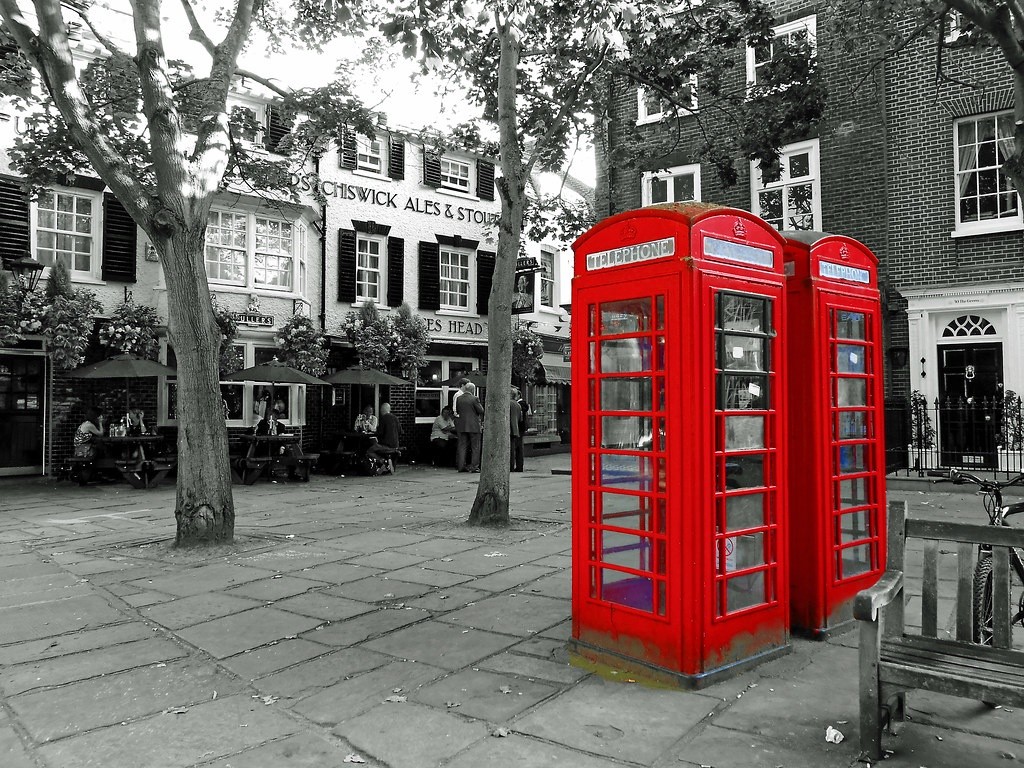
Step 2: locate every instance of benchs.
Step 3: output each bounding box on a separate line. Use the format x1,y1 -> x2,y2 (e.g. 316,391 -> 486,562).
278,452 -> 320,483
67,456 -> 98,485
854,500 -> 1024,764
117,455 -> 174,487
380,445 -> 407,473
322,449 -> 354,472
230,456 -> 273,485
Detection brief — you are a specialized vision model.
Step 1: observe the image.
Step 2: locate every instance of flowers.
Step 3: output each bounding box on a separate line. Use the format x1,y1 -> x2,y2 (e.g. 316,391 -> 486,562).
0,274 -> 173,372
342,300 -> 431,379
273,302 -> 332,380
511,328 -> 544,390
213,301 -> 240,354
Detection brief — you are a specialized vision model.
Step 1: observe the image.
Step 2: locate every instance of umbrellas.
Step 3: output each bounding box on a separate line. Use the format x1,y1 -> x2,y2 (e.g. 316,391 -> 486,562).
221,355 -> 332,480
71,349 -> 177,482
325,360 -> 414,447
438,370 -> 518,389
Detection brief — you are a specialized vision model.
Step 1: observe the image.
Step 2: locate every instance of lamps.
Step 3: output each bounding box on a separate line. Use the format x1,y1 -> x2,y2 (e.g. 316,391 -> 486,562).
400,368 -> 409,378
8,250 -> 46,294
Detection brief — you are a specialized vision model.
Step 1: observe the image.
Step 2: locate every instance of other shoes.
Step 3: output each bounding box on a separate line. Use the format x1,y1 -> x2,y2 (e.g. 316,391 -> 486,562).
472,467 -> 480,473
87,474 -> 102,485
459,466 -> 469,472
514,467 -> 523,472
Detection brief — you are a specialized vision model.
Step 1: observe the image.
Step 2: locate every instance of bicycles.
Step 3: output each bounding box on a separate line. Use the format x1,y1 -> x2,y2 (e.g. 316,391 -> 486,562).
924,466 -> 1024,707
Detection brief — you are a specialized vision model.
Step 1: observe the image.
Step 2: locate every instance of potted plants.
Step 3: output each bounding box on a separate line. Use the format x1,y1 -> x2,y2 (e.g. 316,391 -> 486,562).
907,392 -> 937,468
997,389 -> 1024,473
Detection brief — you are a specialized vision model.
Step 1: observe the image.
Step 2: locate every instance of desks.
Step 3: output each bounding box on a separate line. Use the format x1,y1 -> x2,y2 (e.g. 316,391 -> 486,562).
331,430 -> 376,450
94,436 -> 163,481
240,433 -> 300,480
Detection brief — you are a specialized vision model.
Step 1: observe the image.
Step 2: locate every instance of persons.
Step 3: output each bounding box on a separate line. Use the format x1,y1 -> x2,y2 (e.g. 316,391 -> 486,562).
456,382 -> 484,473
366,403 -> 404,474
509,388 -> 529,472
354,406 -> 378,434
430,378 -> 470,468
74,409 -> 116,484
253,408 -> 286,483
125,409 -> 156,489
